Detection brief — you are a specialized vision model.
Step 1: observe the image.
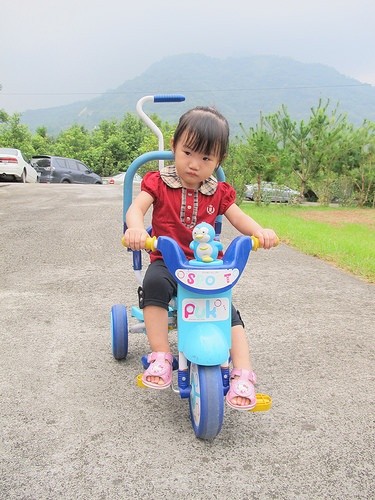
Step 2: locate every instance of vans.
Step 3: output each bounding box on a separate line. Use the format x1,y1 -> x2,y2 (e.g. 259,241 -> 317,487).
27,154 -> 103,185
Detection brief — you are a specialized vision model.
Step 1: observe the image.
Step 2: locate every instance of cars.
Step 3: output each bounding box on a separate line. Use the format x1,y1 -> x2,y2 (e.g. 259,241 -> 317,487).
100,172 -> 142,185
0,147 -> 40,183
244,180 -> 305,203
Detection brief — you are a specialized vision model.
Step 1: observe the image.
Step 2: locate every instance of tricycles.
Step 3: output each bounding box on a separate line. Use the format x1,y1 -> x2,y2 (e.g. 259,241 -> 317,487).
107,93 -> 273,441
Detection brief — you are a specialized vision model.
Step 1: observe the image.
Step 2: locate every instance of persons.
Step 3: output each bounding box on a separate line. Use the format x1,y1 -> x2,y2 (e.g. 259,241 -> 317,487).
123,107 -> 276,411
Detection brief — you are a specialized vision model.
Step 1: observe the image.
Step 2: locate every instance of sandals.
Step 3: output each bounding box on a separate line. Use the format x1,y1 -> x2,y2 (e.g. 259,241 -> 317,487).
141,350 -> 174,389
225,367 -> 257,411
